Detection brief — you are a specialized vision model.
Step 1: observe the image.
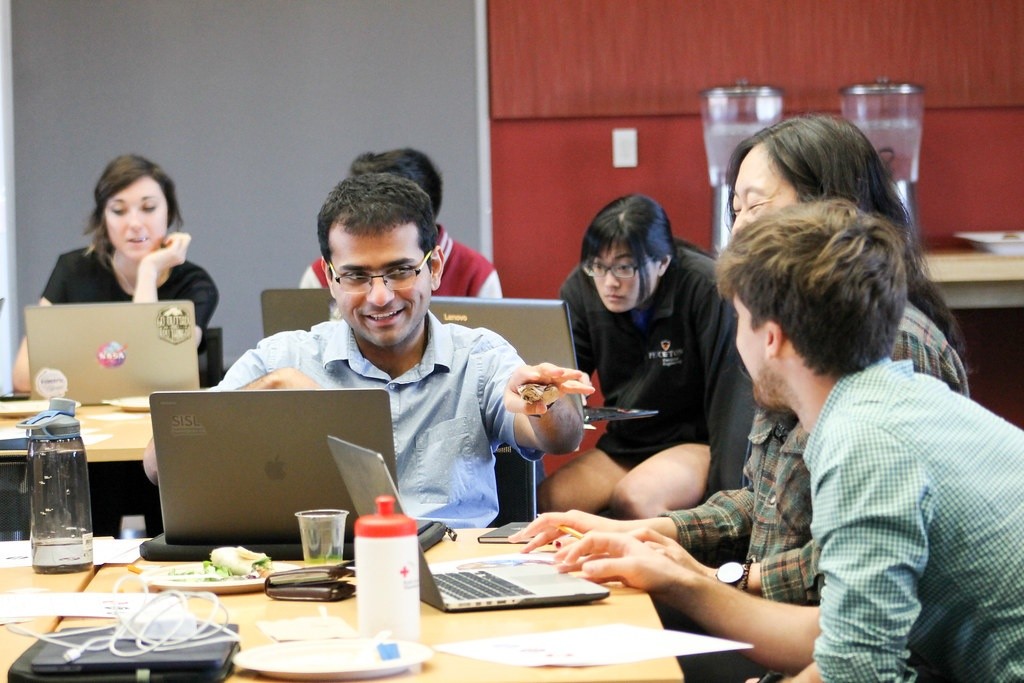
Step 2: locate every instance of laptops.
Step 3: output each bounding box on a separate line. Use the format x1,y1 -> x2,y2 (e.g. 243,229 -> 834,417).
24,300 -> 200,406
149,390 -> 432,541
428,296 -> 659,424
31,624 -> 239,675
260,288 -> 336,339
326,435 -> 609,611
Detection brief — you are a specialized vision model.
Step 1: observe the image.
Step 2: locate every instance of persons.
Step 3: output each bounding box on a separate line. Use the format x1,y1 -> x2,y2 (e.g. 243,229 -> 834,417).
299,148 -> 503,299
507,118 -> 969,683
12,155 -> 219,537
532,193 -> 751,520
555,198 -> 1023,683
143,172 -> 594,526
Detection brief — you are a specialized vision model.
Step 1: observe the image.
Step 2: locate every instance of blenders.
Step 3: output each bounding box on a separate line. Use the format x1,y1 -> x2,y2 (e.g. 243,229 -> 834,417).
840,77 -> 926,258
698,76 -> 782,259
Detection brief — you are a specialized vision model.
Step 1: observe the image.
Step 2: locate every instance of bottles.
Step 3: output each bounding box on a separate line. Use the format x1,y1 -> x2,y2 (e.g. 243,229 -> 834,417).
353,496 -> 420,641
15,396 -> 93,574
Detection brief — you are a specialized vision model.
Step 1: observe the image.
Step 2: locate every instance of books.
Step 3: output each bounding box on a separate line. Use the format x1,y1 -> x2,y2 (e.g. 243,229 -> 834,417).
478,522 -> 530,544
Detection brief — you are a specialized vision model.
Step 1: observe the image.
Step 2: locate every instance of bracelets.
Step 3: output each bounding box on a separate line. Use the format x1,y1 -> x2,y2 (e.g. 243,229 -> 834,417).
741,560 -> 752,589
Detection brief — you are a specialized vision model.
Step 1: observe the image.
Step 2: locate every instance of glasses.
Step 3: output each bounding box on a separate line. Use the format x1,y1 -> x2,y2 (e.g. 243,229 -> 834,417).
328,250 -> 431,294
581,255 -> 655,278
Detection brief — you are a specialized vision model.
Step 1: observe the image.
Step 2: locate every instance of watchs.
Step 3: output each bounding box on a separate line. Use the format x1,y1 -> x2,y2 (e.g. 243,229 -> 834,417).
715,562 -> 743,587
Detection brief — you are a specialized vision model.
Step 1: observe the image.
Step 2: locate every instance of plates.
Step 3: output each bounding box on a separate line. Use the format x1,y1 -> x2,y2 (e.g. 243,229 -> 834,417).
146,563 -> 303,595
232,638 -> 432,680
954,229 -> 1024,256
104,397 -> 150,412
0,400 -> 83,419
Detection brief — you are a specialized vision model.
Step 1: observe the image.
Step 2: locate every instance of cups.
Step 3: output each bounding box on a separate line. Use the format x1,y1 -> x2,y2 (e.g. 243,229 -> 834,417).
295,509 -> 350,567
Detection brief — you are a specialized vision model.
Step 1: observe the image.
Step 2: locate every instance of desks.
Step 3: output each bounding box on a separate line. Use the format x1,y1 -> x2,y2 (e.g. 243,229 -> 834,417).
0,394 -> 153,464
0,527 -> 684,683
924,248 -> 1024,309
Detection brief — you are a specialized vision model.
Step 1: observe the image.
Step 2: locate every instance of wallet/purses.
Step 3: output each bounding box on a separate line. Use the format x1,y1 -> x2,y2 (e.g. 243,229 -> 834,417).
264,566 -> 357,602
